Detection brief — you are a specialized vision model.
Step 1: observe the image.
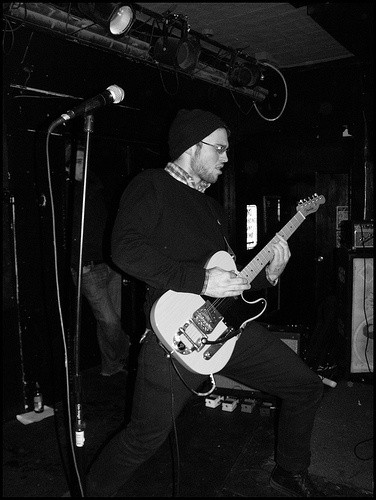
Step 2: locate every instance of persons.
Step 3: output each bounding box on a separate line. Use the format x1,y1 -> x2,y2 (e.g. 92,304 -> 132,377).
54,138 -> 140,389
85,109 -> 324,498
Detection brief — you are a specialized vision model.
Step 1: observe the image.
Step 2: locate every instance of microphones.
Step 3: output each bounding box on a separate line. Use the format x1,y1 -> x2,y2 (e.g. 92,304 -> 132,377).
56,85 -> 126,125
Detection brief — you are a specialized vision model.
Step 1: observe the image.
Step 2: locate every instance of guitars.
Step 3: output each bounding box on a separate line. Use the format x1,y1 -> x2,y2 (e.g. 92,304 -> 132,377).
150,193 -> 326,375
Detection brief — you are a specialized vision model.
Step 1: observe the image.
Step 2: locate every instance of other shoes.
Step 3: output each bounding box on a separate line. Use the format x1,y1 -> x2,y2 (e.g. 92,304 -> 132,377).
112,370 -> 127,384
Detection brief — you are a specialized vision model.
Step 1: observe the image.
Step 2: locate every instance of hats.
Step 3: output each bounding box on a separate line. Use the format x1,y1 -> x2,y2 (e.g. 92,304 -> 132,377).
168,109 -> 225,163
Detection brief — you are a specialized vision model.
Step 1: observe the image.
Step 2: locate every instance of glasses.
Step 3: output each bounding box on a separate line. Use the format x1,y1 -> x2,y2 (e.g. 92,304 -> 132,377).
199,140 -> 230,154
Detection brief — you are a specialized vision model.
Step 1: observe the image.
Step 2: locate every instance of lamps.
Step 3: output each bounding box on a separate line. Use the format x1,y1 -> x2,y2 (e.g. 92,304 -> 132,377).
148,13 -> 197,72
73,0 -> 136,39
226,50 -> 261,89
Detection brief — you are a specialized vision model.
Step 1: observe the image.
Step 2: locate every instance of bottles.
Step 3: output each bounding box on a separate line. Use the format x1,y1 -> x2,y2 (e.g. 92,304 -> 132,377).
33,382 -> 44,413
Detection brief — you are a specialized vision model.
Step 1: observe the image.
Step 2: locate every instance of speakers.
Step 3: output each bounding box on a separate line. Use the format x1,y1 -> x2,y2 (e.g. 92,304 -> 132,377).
345,249 -> 374,379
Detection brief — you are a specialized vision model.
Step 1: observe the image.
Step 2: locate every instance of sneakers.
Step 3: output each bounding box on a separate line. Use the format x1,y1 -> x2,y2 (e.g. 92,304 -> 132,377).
269,464 -> 322,497
